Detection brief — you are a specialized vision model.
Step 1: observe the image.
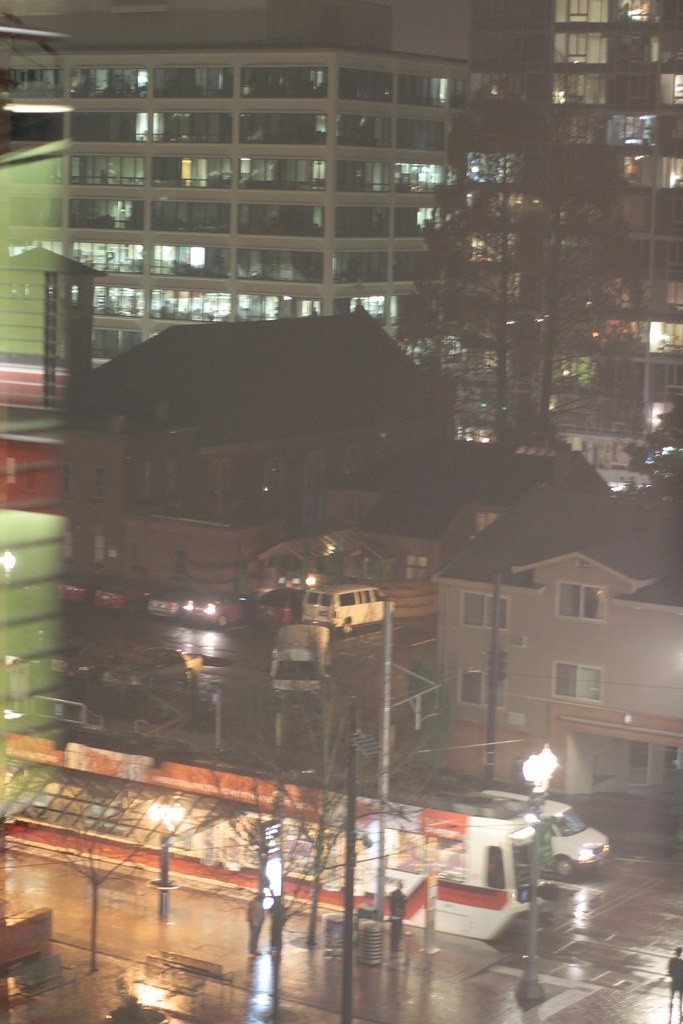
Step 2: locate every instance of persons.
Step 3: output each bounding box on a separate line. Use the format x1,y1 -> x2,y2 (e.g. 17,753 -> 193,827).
268,896 -> 284,955
668,947 -> 683,1024
247,892 -> 265,959
389,881 -> 407,952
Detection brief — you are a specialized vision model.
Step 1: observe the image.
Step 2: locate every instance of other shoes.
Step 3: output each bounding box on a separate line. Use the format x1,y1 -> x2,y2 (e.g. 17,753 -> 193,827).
248,952 -> 261,959
391,946 -> 402,952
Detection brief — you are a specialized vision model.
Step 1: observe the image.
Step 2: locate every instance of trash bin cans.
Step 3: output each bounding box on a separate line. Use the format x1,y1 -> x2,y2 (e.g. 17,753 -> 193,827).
358,919 -> 384,965
354,902 -> 376,948
320,913 -> 349,960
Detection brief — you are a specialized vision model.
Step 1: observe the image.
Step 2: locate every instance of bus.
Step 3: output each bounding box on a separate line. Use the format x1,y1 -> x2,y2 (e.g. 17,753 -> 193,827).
4,733 -> 538,941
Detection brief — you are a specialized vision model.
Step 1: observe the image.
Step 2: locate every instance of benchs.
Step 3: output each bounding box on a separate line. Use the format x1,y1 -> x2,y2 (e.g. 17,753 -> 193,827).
159,949 -> 234,998
9,954 -> 76,1006
130,953 -> 207,1013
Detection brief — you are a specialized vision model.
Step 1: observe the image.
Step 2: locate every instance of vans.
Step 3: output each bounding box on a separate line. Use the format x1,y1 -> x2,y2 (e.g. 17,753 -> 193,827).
302,584 -> 396,636
455,785 -> 609,877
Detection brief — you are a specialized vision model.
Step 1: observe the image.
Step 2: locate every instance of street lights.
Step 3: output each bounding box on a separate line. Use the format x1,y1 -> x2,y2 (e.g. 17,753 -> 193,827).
146,797 -> 183,886
514,741 -> 559,1007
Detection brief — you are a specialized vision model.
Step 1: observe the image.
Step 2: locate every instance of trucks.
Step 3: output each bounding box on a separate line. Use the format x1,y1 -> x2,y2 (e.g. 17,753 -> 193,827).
268,624 -> 334,707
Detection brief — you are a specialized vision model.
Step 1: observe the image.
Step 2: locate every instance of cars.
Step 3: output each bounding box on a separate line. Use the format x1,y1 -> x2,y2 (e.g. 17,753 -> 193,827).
58,573 -> 303,628
100,645 -> 205,692
